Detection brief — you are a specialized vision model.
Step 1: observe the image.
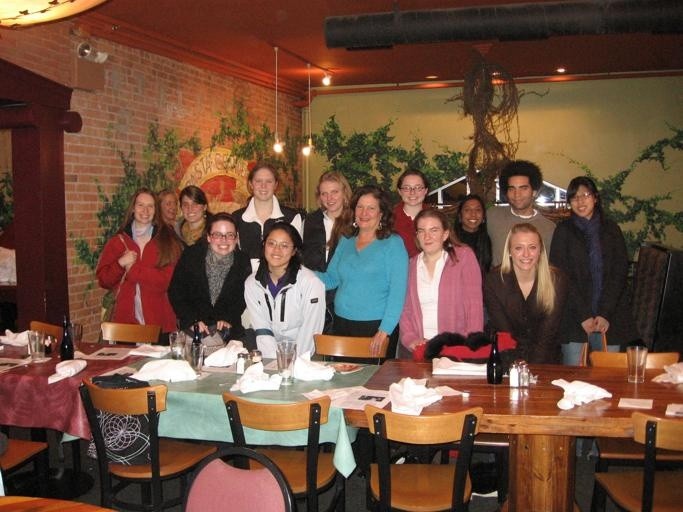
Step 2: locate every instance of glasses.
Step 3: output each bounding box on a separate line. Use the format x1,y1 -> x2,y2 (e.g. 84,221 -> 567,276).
570,191 -> 598,200
207,231 -> 236,240
264,239 -> 294,252
399,184 -> 426,193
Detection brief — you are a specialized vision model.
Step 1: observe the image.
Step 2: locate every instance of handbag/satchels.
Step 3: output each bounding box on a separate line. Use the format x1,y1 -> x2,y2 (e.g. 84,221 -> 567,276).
97,291 -> 114,345
175,324 -> 226,359
83,367 -> 161,465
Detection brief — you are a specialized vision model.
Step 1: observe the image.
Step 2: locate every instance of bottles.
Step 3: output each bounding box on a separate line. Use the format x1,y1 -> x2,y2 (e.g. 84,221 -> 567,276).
59,316 -> 75,360
510,388 -> 529,414
192,324 -> 203,367
237,351 -> 263,374
487,334 -> 503,385
508,359 -> 529,387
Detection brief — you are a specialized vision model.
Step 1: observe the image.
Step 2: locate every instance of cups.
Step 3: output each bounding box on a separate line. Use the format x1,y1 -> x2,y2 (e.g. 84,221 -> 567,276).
626,346 -> 648,383
28,329 -> 46,363
275,341 -> 298,386
168,331 -> 186,358
44,335 -> 55,354
66,322 -> 82,350
183,343 -> 205,370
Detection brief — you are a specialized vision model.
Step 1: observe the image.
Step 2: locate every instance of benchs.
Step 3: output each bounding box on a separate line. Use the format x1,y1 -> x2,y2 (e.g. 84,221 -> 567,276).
612,240 -> 681,355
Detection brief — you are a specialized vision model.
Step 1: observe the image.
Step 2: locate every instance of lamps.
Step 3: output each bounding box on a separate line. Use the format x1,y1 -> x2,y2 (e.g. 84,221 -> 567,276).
268,46 -> 332,158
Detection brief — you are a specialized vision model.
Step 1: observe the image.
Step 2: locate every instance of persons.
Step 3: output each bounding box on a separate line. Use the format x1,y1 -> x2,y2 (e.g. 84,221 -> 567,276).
453,194 -> 493,271
232,161 -> 303,258
393,167 -> 435,259
304,186 -> 409,365
244,223 -> 326,362
95,162 -> 253,348
305,170 -> 354,336
549,175 -> 630,465
398,206 -> 485,361
485,159 -> 557,273
482,221 -> 569,504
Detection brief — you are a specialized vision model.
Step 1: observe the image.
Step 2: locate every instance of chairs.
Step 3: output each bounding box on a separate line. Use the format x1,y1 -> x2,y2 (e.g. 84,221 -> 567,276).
0,320 -> 683,512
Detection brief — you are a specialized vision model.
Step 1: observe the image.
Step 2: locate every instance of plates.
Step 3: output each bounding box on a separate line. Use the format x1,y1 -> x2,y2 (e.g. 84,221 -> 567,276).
324,362 -> 364,376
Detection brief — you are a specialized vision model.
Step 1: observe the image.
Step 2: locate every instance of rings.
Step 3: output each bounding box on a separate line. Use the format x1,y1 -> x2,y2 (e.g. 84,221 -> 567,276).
375,343 -> 379,346
